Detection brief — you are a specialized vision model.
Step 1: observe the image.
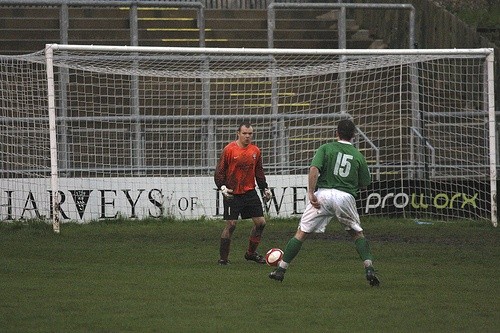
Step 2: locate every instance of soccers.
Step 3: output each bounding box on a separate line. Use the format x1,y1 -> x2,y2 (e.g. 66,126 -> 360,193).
264,248 -> 285,267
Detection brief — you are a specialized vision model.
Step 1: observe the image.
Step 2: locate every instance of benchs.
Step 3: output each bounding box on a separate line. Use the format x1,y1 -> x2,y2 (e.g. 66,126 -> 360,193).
0,7 -> 488,178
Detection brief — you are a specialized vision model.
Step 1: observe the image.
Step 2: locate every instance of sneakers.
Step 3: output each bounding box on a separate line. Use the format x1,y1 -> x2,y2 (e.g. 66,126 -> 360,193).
244,252 -> 266,264
218,258 -> 230,264
366,270 -> 380,287
269,269 -> 284,280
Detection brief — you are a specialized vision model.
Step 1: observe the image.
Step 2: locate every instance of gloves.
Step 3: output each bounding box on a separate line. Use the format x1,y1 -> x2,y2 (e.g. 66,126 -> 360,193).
262,188 -> 272,199
220,185 -> 233,201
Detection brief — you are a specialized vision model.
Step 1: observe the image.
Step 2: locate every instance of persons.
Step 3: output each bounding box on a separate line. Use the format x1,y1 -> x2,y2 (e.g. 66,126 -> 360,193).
214,122 -> 272,266
268,120 -> 379,287
